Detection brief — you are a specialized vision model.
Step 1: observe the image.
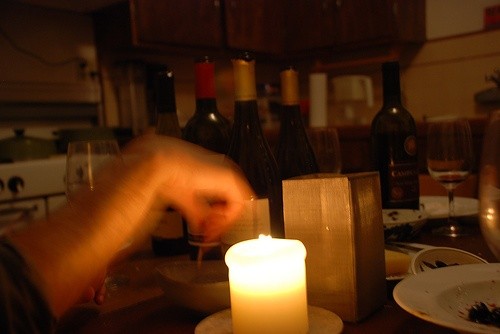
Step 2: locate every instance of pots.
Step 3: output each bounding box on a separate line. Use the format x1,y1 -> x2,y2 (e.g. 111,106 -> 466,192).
51,126 -> 135,155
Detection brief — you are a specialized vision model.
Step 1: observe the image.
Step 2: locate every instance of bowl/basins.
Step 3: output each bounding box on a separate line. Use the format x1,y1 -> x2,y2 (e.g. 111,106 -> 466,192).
155,260 -> 232,314
382,209 -> 430,241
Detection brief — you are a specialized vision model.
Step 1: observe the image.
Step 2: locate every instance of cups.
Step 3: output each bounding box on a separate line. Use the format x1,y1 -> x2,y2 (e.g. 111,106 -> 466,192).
478,110 -> 500,259
306,127 -> 341,174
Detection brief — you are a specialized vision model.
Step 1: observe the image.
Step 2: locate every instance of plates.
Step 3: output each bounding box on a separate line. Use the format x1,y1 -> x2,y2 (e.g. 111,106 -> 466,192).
412,246 -> 489,277
194,305 -> 344,334
392,263 -> 500,334
385,242 -> 438,280
419,195 -> 479,219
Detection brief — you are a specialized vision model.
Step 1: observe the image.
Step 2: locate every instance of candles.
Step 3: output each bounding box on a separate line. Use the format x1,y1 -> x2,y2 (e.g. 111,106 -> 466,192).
224,231 -> 308,334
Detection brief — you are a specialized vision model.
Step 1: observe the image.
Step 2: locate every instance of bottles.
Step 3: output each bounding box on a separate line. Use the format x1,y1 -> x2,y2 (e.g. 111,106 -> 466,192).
371,61 -> 420,210
144,56 -> 321,261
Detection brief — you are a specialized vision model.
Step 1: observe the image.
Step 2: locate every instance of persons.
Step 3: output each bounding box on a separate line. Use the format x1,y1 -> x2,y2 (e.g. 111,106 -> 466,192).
0,135 -> 252,334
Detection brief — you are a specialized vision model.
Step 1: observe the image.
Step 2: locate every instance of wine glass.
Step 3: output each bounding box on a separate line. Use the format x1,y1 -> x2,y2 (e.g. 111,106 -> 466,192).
65,139 -> 123,288
424,119 -> 474,237
